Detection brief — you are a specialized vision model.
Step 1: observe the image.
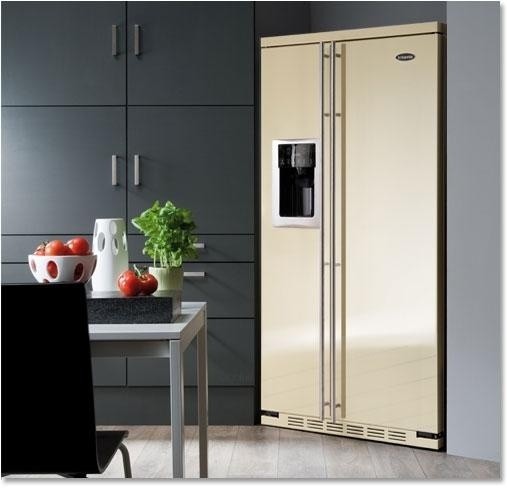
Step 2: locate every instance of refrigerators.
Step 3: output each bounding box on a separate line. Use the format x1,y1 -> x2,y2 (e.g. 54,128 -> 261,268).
258,20 -> 447,456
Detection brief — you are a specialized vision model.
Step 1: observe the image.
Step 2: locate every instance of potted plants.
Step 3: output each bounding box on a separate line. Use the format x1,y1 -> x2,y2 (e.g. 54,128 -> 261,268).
129,200 -> 201,316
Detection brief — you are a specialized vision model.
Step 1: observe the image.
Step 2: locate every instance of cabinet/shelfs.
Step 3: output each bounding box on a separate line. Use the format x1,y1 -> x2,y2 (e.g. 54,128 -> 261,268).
1,103 -> 258,236
0,233 -> 260,425
2,0 -> 259,106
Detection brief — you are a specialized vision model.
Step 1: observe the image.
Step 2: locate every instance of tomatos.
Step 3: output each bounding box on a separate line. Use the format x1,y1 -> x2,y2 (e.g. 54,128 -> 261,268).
138,274 -> 158,296
118,270 -> 138,296
33,237 -> 93,280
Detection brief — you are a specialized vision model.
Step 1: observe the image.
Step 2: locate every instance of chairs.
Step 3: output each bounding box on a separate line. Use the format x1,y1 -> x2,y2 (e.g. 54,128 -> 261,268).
3,281 -> 133,478
